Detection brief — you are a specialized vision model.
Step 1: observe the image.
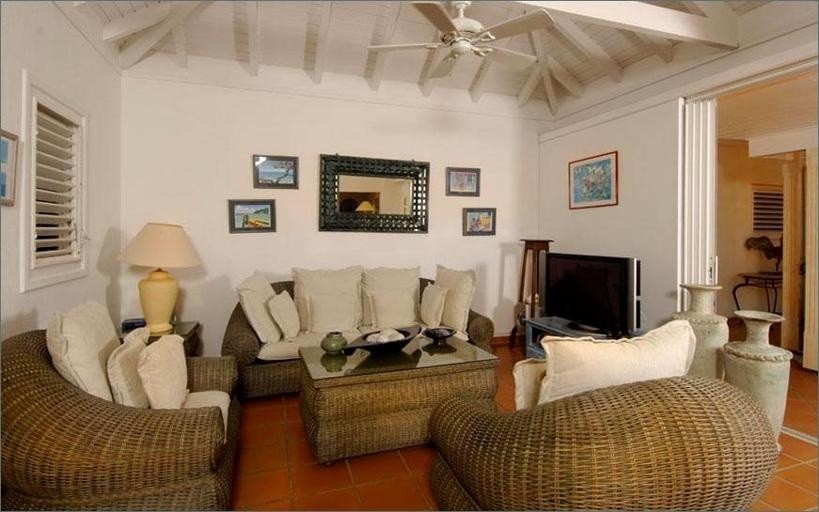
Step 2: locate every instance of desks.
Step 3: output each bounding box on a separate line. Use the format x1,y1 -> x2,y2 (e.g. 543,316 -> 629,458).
733,272 -> 783,327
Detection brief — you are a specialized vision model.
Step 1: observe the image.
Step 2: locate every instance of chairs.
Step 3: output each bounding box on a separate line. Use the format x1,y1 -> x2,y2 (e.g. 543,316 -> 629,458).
429,373 -> 779,511
1,322 -> 240,511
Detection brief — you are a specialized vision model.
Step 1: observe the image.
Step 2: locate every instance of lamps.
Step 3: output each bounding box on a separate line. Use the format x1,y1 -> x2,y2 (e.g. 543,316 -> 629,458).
115,222 -> 203,337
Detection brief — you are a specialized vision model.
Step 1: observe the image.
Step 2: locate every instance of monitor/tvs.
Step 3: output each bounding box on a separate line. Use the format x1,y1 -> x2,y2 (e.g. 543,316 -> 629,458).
544,251 -> 630,336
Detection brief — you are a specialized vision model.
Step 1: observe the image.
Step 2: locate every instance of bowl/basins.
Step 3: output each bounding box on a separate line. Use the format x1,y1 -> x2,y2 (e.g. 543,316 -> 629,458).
352,343 -> 422,372
344,325 -> 421,356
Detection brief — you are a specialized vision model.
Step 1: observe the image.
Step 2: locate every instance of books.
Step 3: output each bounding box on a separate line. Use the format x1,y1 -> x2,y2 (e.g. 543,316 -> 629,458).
122,318 -> 144,327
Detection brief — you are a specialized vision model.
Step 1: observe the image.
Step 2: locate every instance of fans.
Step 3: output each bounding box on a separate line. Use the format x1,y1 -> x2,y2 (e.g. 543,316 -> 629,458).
366,0 -> 555,80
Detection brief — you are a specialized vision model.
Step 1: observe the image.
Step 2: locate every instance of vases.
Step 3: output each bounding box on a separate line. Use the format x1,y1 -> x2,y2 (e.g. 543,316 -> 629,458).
723,309 -> 792,454
671,283 -> 730,379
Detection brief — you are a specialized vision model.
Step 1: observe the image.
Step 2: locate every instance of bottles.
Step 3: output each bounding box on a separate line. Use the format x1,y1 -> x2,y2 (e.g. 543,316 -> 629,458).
319,329 -> 348,356
319,354 -> 346,372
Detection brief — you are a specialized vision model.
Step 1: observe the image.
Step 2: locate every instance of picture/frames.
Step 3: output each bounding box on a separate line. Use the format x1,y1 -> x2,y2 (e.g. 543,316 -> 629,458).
462,207 -> 498,236
446,166 -> 480,197
569,150 -> 619,210
0,128 -> 19,207
228,198 -> 276,234
253,154 -> 300,190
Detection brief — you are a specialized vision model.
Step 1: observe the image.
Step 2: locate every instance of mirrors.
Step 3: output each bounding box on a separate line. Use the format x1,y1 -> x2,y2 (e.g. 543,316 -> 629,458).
318,153 -> 431,234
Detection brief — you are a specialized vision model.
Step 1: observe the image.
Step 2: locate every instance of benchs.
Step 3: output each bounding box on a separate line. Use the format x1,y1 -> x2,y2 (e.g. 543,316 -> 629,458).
222,278 -> 494,398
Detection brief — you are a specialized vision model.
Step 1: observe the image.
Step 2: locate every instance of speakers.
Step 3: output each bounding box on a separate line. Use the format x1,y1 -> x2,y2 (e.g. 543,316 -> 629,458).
629,256 -> 641,332
539,249 -> 547,307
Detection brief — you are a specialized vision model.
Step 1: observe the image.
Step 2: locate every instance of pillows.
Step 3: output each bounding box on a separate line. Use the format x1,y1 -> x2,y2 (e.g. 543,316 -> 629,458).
236,264 -> 479,345
45,301 -> 189,410
511,318 -> 697,411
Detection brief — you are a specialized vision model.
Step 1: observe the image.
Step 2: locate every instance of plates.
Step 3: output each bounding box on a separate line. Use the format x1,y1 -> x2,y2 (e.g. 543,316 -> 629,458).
421,328 -> 457,341
421,342 -> 457,354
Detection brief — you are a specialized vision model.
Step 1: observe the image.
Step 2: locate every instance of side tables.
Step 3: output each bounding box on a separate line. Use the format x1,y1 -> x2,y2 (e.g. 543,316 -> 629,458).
119,321 -> 201,358
509,239 -> 555,347
